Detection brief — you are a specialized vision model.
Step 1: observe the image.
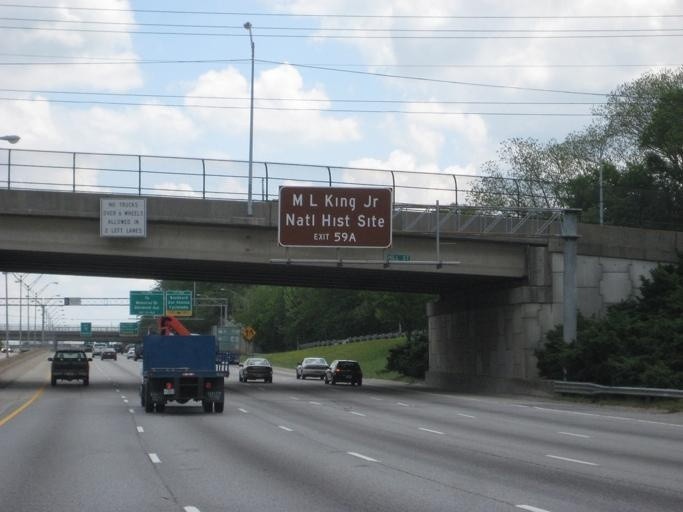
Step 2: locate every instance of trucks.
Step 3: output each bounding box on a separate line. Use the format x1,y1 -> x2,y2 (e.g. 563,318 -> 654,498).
141,336 -> 229,413
209,325 -> 242,365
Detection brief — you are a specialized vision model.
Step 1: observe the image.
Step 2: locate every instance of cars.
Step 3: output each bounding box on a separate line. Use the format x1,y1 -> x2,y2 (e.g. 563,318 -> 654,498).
47,350 -> 93,385
85,342 -> 144,361
240,357 -> 273,383
297,357 -> 362,385
1,345 -> 13,353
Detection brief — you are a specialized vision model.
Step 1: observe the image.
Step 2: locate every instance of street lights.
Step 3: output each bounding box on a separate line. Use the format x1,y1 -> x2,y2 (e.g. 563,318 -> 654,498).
220,288 -> 250,312
243,22 -> 254,217
34,282 -> 68,345
0,135 -> 21,144
197,293 -> 223,326
